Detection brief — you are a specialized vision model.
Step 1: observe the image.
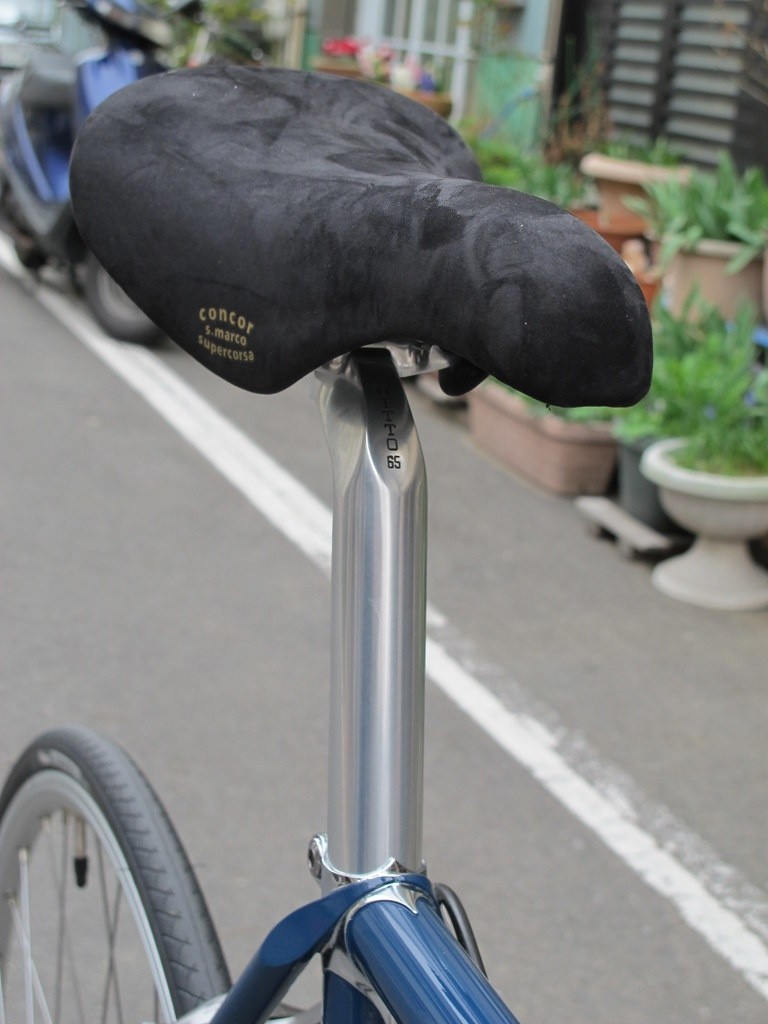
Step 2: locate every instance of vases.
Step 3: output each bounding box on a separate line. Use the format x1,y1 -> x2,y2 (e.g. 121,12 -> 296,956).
310,58 -> 359,75
398,87 -> 453,119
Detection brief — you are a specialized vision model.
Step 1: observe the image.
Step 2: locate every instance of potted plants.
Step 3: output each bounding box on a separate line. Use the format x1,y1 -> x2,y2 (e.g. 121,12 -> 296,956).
577,131 -> 698,234
465,374 -> 617,497
623,146 -> 768,328
611,282 -> 756,533
637,393 -> 768,612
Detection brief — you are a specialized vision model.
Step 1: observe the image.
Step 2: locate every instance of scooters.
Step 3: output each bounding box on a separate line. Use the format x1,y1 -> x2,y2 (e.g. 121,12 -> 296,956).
0,0 -> 219,348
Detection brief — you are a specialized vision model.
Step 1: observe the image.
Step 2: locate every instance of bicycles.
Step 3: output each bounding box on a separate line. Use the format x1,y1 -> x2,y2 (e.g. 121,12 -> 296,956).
1,61 -> 662,1024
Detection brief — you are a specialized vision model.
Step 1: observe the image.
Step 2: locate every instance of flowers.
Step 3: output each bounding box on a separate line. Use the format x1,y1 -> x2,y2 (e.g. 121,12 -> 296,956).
322,33 -> 366,68
357,44 -> 395,82
404,53 -> 444,93
